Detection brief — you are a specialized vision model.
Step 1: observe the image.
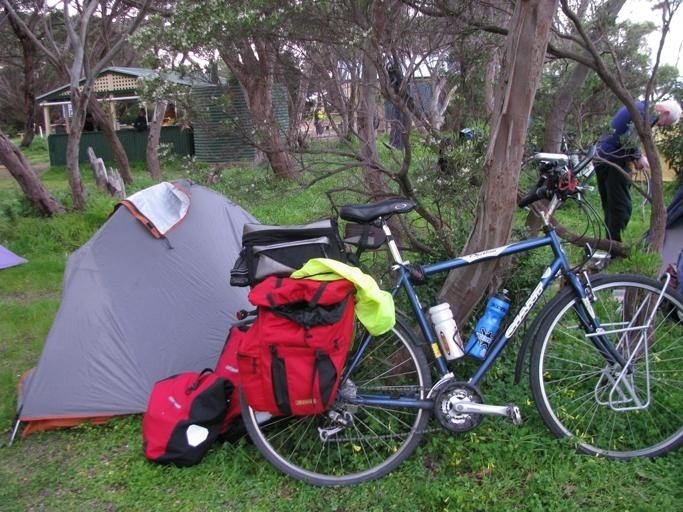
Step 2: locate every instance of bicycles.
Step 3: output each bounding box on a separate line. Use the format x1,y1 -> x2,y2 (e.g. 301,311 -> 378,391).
232,154 -> 683,488
516,141 -> 651,210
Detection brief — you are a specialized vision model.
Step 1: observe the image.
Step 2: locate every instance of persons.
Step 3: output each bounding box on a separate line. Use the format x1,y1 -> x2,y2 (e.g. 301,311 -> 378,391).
312,104 -> 326,135
592,99 -> 680,257
133,109 -> 147,130
161,104 -> 175,122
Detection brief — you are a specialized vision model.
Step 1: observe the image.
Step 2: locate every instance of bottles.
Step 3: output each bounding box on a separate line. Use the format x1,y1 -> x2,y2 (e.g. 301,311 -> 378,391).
427,302 -> 466,361
463,288 -> 512,361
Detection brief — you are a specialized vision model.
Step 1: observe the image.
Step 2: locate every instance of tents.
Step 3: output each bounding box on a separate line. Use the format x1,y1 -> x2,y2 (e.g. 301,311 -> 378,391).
14,178 -> 262,435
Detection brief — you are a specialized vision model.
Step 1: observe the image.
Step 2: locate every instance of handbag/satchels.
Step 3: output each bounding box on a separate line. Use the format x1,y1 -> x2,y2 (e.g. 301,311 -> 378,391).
140,371 -> 237,464
213,216 -> 354,438
